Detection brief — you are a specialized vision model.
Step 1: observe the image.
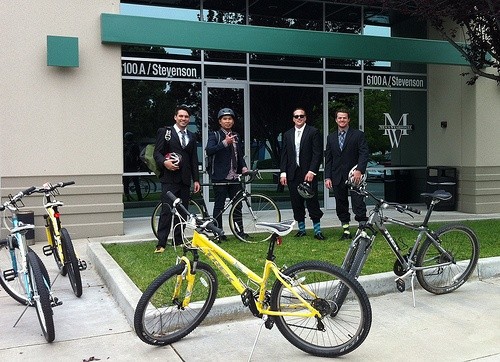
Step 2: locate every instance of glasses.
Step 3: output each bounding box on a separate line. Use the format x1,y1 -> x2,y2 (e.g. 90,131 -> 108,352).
293,115 -> 306,118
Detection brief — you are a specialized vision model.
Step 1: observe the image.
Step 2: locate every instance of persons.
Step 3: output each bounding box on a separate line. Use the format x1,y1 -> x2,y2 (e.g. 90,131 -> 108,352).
279,108 -> 327,239
205,108 -> 251,241
324,108 -> 370,240
124,132 -> 146,201
152,106 -> 200,253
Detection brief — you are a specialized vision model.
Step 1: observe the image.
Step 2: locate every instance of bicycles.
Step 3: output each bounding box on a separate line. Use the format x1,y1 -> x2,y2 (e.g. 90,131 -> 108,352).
329,181 -> 480,317
152,170 -> 281,246
133,190 -> 372,361
0,186 -> 63,343
29,181 -> 88,297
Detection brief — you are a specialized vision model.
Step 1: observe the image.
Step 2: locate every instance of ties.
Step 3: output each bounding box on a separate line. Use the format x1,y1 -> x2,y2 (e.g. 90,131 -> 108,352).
232,144 -> 237,169
339,132 -> 346,152
181,131 -> 187,149
296,131 -> 301,167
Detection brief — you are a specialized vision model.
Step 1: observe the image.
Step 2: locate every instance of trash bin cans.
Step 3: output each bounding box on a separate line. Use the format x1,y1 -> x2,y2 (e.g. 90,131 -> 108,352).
426,164 -> 458,212
15,209 -> 35,246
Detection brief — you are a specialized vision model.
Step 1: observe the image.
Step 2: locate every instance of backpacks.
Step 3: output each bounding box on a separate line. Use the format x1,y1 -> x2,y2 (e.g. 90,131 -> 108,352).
145,143 -> 161,179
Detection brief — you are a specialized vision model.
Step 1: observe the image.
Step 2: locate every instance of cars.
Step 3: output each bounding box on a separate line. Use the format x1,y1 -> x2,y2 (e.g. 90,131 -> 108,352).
366,161 -> 391,180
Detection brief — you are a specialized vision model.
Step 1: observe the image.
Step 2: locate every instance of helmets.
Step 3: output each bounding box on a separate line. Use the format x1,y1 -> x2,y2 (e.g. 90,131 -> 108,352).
348,164 -> 368,189
296,181 -> 314,199
164,153 -> 183,170
218,107 -> 235,119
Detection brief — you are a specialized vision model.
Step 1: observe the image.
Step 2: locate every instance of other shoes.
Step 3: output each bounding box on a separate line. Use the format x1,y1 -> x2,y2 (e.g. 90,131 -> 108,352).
294,231 -> 306,237
240,234 -> 248,239
343,228 -> 352,239
314,233 -> 325,240
153,246 -> 165,253
